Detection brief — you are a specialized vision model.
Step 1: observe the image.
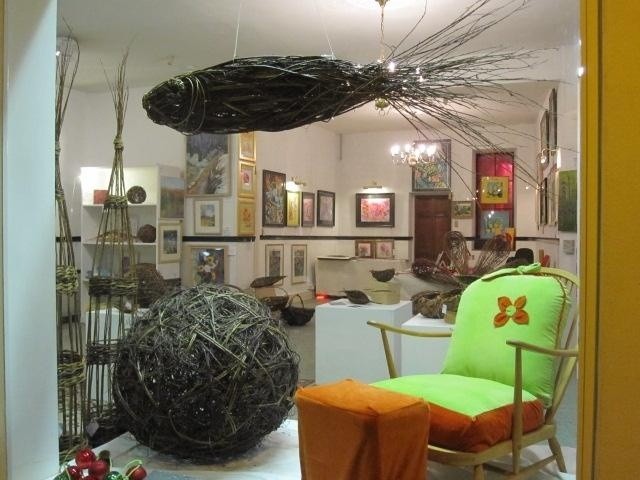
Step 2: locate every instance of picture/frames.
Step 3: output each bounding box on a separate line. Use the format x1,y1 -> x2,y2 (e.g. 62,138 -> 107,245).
237,161 -> 258,198
262,169 -> 286,227
291,244 -> 308,284
188,243 -> 229,285
548,87 -> 557,156
355,193 -> 395,228
238,133 -> 256,162
479,175 -> 509,204
539,177 -> 547,226
159,223 -> 182,264
287,189 -> 300,227
557,170 -> 578,233
236,199 -> 256,236
317,254 -> 353,260
183,134 -> 232,197
451,200 -> 474,219
478,210 -> 510,240
412,139 -> 451,191
375,240 -> 394,260
265,244 -> 285,288
301,192 -> 315,227
159,174 -> 185,220
193,199 -> 223,236
355,241 -> 373,258
317,190 -> 336,228
539,109 -> 549,170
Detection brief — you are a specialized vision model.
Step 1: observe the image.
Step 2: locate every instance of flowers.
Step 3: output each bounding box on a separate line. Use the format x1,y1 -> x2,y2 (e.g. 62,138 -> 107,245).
52,437 -> 148,480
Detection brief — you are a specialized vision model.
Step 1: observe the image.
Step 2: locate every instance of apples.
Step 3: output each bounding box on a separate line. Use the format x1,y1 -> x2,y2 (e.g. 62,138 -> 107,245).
126,466 -> 147,480
67,450 -> 107,480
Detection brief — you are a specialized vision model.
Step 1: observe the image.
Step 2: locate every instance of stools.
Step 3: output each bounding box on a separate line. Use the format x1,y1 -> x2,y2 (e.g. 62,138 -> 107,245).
296,379 -> 431,480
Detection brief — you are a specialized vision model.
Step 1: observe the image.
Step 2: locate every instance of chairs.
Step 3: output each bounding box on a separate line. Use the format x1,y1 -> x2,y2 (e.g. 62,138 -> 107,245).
366,265 -> 578,480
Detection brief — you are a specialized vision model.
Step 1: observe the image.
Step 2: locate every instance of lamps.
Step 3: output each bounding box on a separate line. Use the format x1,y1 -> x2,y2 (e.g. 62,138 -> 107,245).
540,147 -> 558,163
387,123 -> 439,169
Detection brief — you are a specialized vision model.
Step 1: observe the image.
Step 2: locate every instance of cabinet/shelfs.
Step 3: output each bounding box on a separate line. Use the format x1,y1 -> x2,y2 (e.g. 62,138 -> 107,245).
86,306 -> 148,405
69,416 -> 303,480
79,164 -> 181,315
315,301 -> 412,381
401,304 -> 455,376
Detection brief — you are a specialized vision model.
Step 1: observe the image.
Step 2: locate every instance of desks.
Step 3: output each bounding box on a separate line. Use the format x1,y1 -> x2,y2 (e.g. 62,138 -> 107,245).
315,256 -> 408,297
